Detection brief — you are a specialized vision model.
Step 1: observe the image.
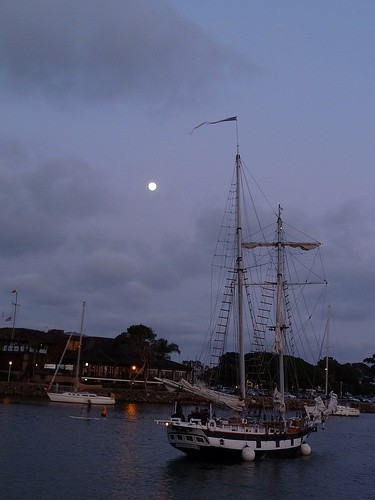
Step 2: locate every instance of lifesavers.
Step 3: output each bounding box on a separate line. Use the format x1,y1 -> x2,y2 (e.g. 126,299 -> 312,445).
207,419 -> 217,432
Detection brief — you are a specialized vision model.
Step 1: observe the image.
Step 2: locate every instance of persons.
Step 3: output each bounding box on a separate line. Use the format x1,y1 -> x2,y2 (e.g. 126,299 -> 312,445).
187,407 -> 210,425
86,400 -> 93,418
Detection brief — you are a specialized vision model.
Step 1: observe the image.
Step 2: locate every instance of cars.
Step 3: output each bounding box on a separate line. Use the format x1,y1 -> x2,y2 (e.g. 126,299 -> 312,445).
214,386 -> 375,410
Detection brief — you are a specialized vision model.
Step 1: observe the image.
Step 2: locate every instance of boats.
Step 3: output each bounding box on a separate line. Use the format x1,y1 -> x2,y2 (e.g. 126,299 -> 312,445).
305,405 -> 361,416
50,391 -> 117,405
165,156 -> 334,460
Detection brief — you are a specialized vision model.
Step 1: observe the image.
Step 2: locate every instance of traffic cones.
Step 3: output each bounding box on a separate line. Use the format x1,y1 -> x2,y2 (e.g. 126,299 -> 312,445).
102,405 -> 107,417
